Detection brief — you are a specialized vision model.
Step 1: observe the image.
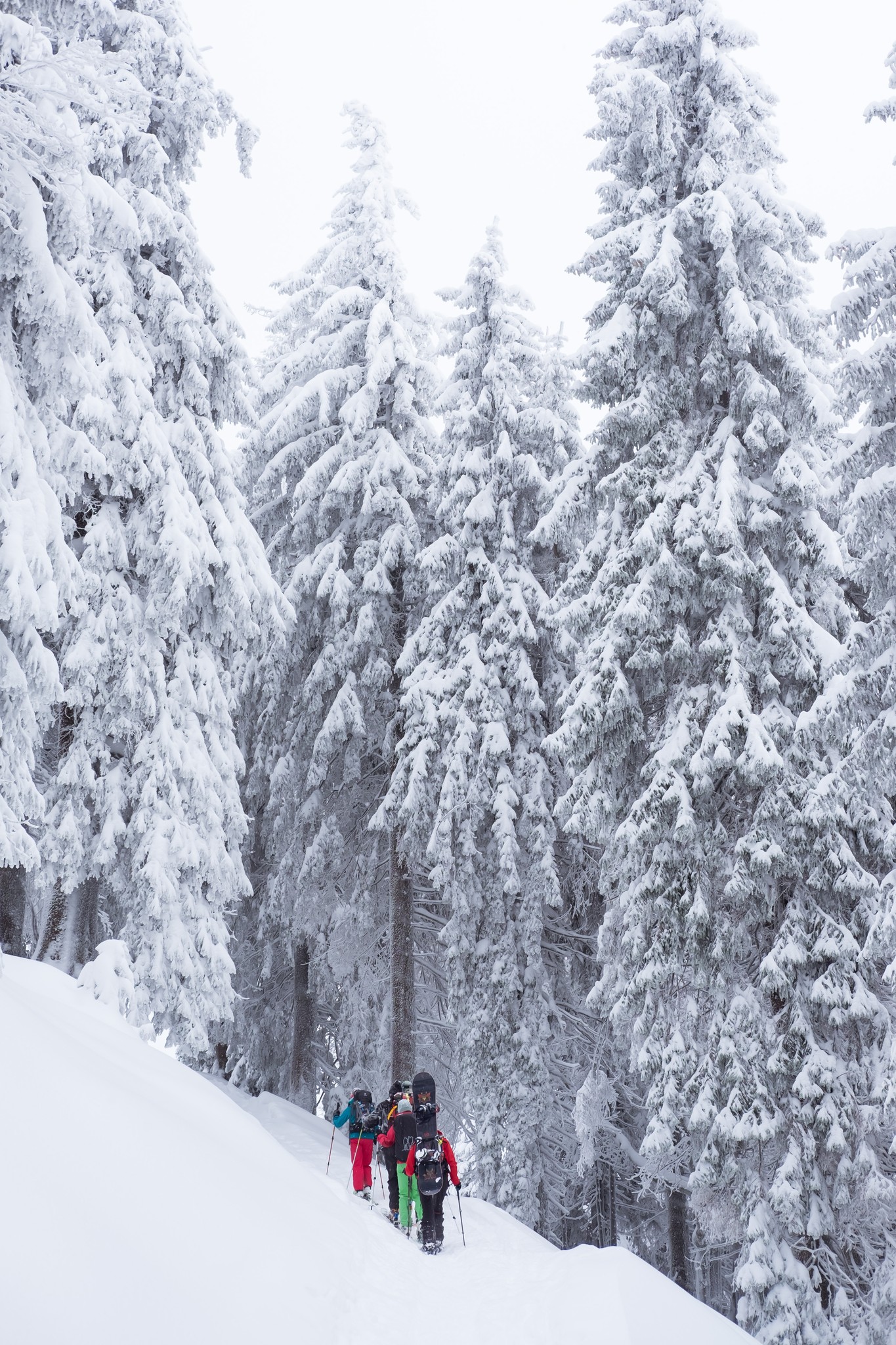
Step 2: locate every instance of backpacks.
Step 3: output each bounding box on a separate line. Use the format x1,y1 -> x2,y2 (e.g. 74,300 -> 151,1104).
393,1114 -> 416,1162
350,1090 -> 374,1133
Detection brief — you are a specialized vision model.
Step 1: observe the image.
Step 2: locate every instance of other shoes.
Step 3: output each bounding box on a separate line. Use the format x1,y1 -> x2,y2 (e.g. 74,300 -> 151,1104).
435,1240 -> 442,1252
353,1190 -> 363,1197
364,1186 -> 371,1197
422,1243 -> 436,1255
388,1209 -> 398,1219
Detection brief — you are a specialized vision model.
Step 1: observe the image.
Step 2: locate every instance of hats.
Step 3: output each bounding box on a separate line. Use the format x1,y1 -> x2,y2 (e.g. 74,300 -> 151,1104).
398,1099 -> 413,1113
389,1079 -> 403,1095
351,1087 -> 361,1097
401,1080 -> 414,1094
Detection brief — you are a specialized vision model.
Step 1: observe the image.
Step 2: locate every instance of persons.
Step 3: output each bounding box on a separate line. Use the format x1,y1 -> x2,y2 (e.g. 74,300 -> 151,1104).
333,1087 -> 375,1198
402,1128 -> 461,1254
361,1079 -> 440,1237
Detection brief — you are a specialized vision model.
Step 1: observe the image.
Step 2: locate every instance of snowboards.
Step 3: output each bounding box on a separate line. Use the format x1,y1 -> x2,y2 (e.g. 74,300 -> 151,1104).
412,1072 -> 443,1195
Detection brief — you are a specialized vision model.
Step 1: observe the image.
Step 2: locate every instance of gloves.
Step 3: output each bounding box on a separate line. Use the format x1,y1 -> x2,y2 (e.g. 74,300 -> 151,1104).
333,1110 -> 339,1117
374,1129 -> 382,1137
402,1168 -> 407,1175
455,1182 -> 461,1191
